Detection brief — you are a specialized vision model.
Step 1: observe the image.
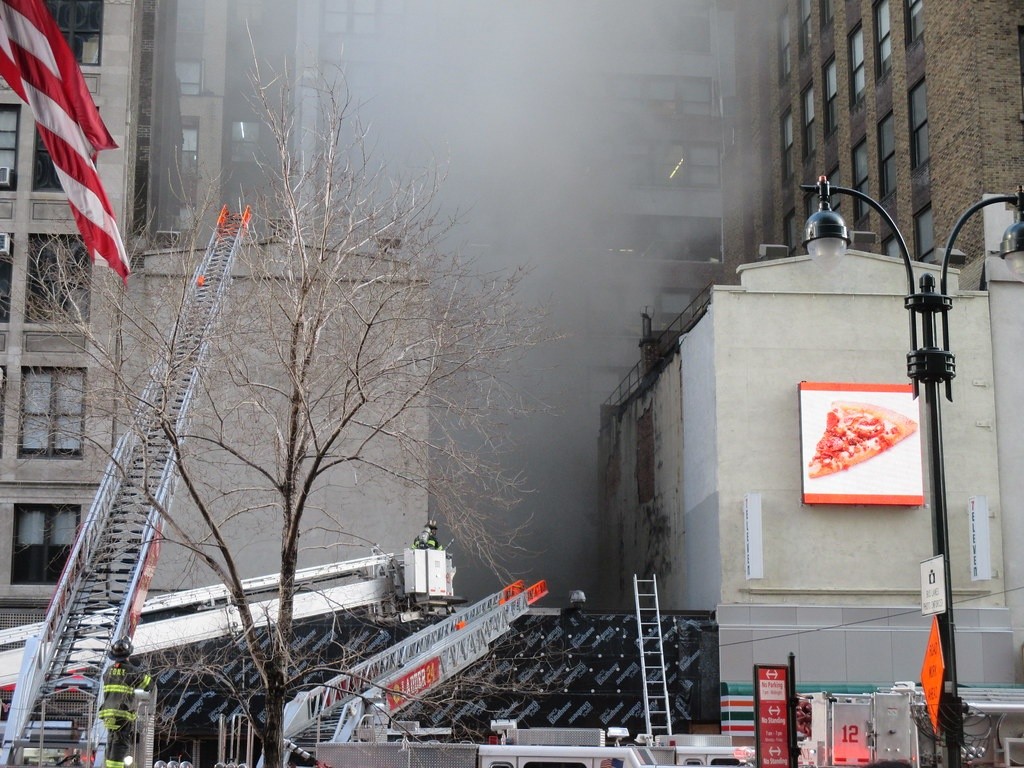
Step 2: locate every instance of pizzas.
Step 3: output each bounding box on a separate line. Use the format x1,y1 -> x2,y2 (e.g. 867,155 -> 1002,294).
808,399 -> 919,478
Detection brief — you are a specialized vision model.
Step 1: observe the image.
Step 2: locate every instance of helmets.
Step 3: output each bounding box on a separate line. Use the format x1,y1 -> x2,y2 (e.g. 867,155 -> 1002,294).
570,590 -> 586,603
108,640 -> 134,661
425,520 -> 437,530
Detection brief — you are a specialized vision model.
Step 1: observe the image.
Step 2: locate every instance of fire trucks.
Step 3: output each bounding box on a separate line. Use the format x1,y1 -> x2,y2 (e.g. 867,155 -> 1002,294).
0,201 -> 1024,767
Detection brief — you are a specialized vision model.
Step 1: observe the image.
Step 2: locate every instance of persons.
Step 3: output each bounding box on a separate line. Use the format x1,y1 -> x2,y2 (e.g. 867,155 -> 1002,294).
559,589 -> 598,694
98,639 -> 156,768
411,519 -> 438,550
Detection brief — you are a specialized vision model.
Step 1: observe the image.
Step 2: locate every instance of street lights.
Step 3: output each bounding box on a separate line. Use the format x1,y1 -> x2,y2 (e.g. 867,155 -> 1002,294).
798,175 -> 1024,767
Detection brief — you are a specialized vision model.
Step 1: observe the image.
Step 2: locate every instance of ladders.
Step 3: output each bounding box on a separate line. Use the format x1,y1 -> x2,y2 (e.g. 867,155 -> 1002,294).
282,578 -> 549,739
632,573 -> 673,737
0,202 -> 251,768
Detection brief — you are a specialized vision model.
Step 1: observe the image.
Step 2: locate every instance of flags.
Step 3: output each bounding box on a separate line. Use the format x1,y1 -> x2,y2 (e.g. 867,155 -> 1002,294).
0,0 -> 131,290
